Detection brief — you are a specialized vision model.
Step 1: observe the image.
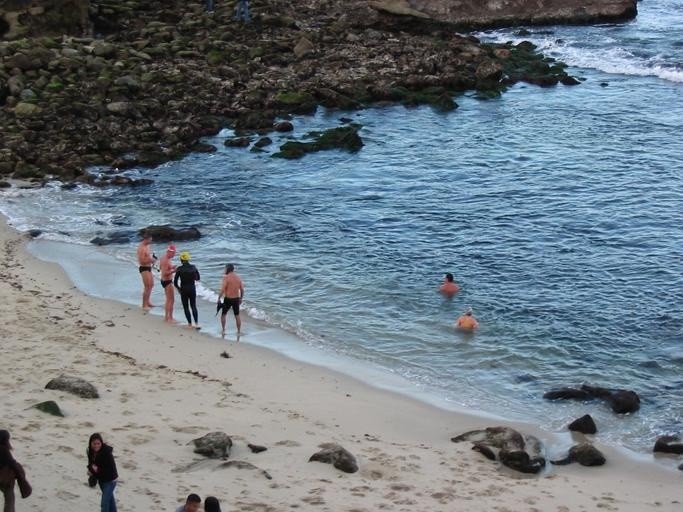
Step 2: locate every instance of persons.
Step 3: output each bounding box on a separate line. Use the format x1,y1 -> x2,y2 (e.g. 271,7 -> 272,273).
203,496 -> 220,512
159,245 -> 177,324
455,307 -> 478,329
217,264 -> 243,335
438,273 -> 458,294
137,231 -> 156,308
86,432 -> 118,512
0,429 -> 24,512
174,494 -> 200,512
173,251 -> 200,329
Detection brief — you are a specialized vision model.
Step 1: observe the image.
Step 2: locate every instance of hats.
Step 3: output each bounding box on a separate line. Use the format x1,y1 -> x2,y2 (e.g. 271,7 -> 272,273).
180,252 -> 190,260
167,245 -> 176,252
